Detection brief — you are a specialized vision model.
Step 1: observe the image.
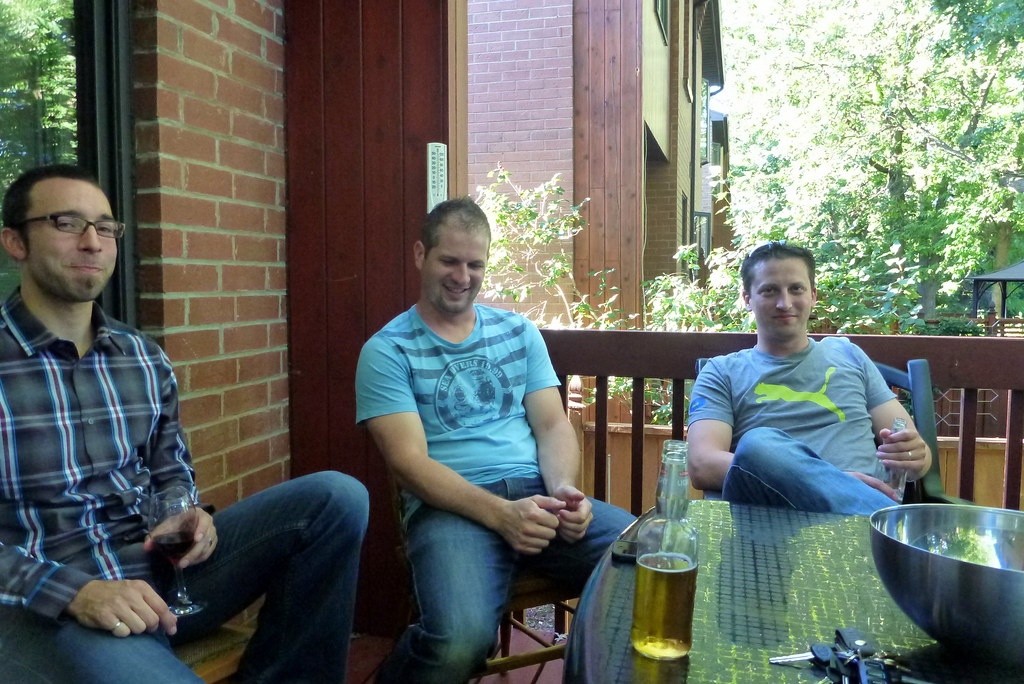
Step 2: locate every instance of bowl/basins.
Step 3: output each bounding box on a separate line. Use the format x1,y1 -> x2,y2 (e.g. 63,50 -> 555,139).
869,504 -> 1024,671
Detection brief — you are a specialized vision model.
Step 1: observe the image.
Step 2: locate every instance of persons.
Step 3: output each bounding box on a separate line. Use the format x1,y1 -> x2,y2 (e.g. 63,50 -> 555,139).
679,242 -> 937,516
0,166 -> 370,684
350,197 -> 643,684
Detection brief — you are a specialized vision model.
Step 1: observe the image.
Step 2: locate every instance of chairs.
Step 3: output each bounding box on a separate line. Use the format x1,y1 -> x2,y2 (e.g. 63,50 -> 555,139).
696,350 -> 984,517
388,468 -> 599,684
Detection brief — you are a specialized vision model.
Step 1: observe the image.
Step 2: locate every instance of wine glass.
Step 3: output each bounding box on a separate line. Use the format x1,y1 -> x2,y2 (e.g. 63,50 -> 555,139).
147,486 -> 205,617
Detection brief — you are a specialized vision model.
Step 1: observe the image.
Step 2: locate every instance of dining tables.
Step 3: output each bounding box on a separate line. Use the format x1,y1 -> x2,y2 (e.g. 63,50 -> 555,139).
559,490 -> 1024,684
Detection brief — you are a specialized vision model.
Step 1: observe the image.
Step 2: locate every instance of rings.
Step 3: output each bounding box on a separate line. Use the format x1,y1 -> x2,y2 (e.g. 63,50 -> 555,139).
907,451 -> 913,461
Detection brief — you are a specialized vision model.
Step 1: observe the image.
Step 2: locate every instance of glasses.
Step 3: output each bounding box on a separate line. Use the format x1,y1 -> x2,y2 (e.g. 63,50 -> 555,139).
15,215 -> 126,238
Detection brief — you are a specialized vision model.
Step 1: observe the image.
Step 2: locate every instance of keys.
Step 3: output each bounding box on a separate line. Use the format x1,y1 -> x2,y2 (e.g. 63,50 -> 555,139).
768,629 -> 932,684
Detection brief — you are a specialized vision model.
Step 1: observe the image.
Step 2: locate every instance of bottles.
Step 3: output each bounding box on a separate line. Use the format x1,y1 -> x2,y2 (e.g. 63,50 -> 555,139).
873,418 -> 908,504
629,440 -> 700,661
